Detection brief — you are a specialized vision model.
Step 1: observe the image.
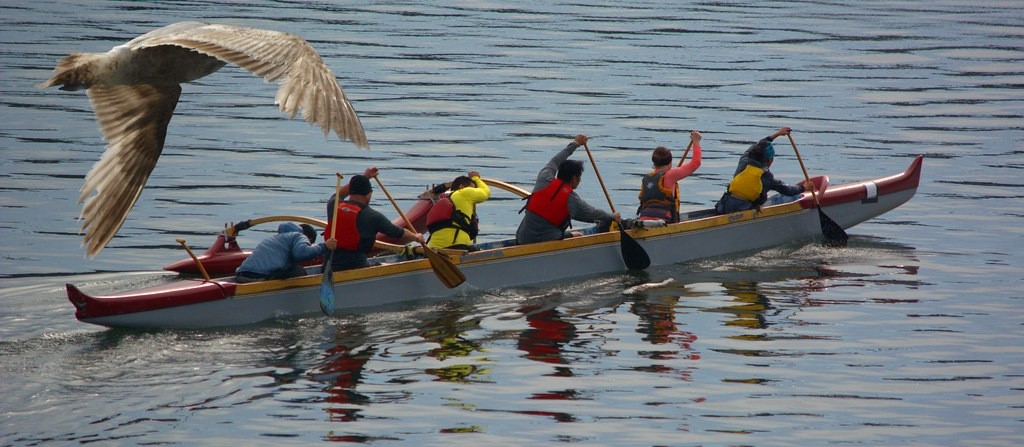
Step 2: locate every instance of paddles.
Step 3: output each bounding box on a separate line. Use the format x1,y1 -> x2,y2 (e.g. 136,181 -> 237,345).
787,132 -> 849,242
175,237 -> 210,280
318,172 -> 345,317
373,175 -> 467,290
584,143 -> 651,270
677,140 -> 694,167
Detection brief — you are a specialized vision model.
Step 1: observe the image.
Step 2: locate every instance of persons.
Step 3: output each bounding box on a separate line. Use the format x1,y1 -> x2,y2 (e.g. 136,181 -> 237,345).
422,170 -> 490,257
715,126 -> 815,219
514,134 -> 621,246
231,225 -> 338,274
324,165 -> 425,270
634,129 -> 702,225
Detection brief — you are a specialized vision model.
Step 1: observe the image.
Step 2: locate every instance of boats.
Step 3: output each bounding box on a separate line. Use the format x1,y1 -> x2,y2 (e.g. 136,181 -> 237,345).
66,154 -> 925,336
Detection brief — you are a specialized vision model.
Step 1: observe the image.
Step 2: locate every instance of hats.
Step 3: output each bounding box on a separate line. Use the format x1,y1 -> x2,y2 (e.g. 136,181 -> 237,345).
349,174 -> 372,194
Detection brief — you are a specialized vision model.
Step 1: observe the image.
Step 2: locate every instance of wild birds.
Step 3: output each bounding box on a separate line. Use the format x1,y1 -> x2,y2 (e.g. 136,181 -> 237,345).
33,22 -> 374,261
